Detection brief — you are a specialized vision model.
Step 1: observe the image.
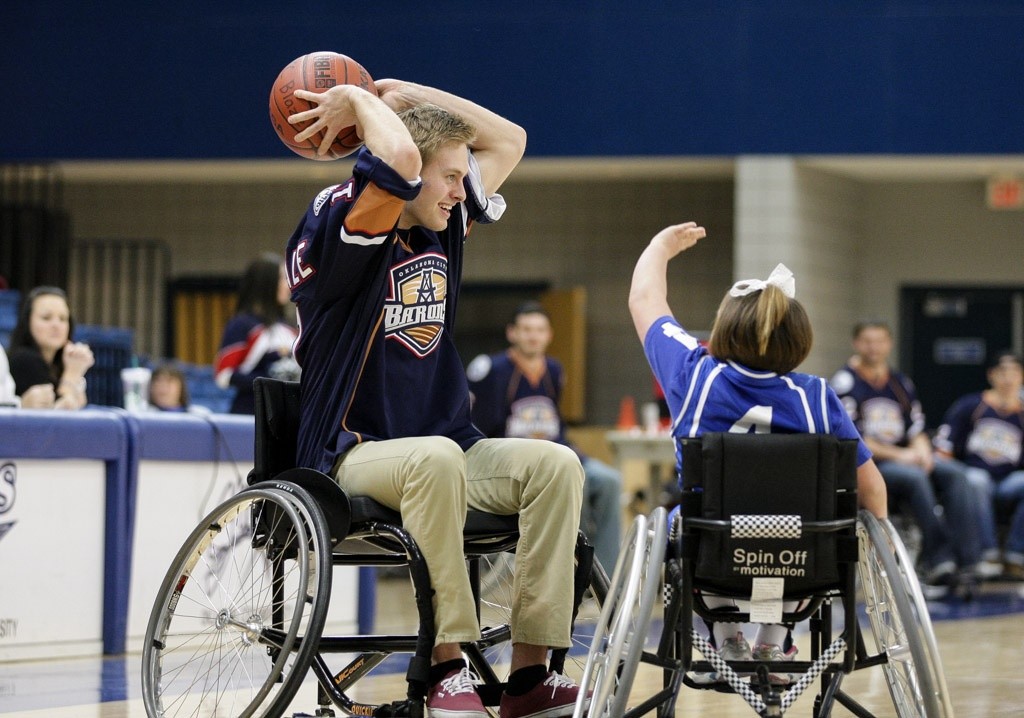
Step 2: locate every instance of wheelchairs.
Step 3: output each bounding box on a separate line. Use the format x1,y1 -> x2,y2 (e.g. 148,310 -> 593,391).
572,432 -> 954,718
141,376 -> 626,718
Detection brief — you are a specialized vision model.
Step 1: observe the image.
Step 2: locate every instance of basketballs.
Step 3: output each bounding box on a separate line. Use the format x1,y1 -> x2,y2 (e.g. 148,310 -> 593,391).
267,49 -> 380,163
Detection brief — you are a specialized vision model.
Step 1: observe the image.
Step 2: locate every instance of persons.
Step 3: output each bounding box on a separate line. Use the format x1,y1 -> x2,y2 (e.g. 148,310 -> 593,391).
629,224 -> 897,685
286,77 -> 592,718
467,301 -> 621,597
214,251 -> 302,414
7,286 -> 94,409
934,352 -> 1024,580
147,359 -> 213,414
828,315 -> 1004,584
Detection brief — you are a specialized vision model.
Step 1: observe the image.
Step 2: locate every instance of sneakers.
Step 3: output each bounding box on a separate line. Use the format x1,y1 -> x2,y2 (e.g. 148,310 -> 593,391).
426,667 -> 489,718
500,670 -> 598,718
709,630 -> 754,682
748,642 -> 802,686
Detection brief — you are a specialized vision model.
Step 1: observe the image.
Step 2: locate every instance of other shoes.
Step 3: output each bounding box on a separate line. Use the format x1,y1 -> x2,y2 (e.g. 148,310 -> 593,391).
925,556 -> 1023,584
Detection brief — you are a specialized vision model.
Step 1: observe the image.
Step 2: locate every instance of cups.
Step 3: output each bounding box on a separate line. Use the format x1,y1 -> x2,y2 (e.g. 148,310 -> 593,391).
121,367 -> 151,415
642,405 -> 659,431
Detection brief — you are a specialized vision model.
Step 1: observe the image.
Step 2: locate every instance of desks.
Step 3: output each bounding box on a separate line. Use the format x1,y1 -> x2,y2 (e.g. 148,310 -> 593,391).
0,404 -> 377,664
